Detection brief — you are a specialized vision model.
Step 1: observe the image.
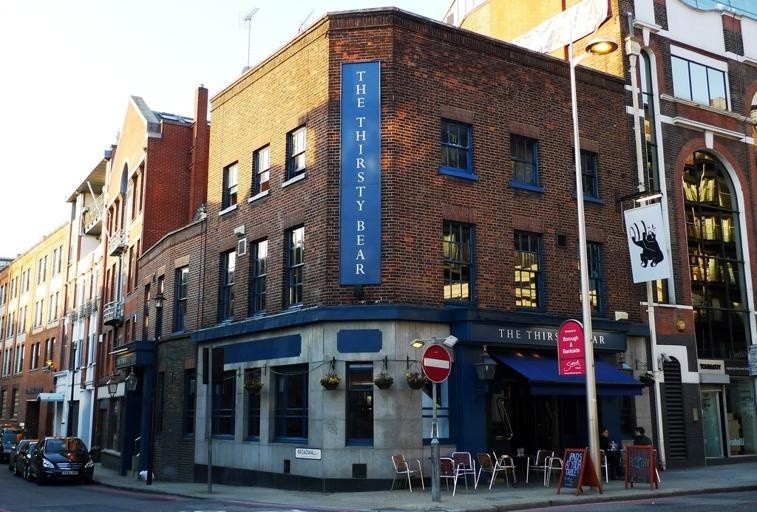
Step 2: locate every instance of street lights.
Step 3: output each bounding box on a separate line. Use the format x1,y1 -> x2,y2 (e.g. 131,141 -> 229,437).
567,40 -> 619,491
408,335 -> 458,501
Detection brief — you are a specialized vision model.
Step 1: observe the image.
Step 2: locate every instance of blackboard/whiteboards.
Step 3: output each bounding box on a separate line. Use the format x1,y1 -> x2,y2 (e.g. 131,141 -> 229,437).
625,445 -> 653,483
559,448 -> 588,488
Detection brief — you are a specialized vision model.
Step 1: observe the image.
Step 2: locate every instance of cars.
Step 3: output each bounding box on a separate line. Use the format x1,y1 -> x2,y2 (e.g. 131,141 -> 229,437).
1,427 -> 94,486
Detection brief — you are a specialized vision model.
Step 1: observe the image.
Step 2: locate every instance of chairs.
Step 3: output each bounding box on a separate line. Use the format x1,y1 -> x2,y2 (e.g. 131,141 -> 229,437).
388,445 -> 661,492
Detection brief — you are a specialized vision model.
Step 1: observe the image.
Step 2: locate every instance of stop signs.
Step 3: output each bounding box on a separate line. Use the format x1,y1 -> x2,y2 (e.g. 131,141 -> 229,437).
421,344 -> 452,383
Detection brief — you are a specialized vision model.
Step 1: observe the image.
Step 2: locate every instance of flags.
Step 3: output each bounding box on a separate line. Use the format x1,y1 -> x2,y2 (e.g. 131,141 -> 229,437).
622,203 -> 673,285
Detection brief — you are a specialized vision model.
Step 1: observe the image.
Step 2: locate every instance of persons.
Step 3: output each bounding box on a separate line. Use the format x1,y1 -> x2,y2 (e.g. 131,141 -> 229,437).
634,426 -> 651,444
598,426 -> 623,480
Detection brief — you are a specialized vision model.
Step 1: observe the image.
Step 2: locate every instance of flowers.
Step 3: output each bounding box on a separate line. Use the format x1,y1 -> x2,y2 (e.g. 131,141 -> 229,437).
241,371 -> 262,394
373,359 -> 393,391
320,361 -> 341,389
405,361 -> 429,391
